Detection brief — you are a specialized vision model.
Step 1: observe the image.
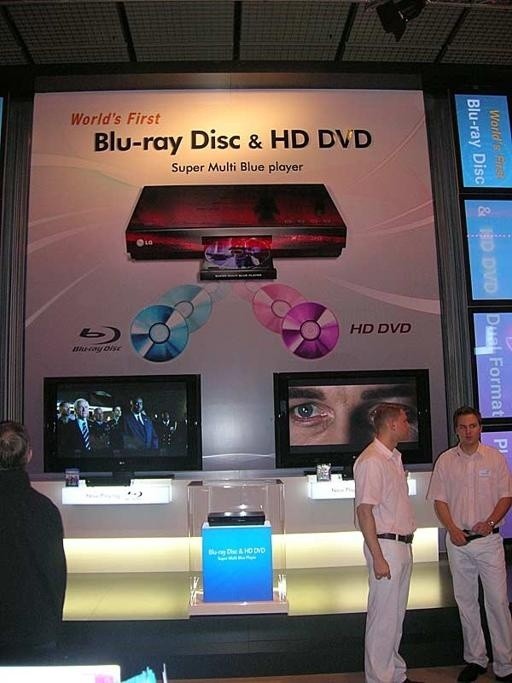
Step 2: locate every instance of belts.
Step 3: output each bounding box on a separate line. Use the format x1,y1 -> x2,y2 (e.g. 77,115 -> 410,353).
461,527 -> 499,543
377,533 -> 413,543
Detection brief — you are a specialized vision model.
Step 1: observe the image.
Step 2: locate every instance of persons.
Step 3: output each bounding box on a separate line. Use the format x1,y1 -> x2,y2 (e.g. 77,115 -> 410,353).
57,397 -> 188,456
427,407 -> 512,681
289,383 -> 418,446
353,406 -> 417,682
0,419 -> 67,665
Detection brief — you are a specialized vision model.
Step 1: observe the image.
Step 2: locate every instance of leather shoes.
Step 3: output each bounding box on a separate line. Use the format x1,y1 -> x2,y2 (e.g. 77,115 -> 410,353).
495,673 -> 512,683
458,663 -> 487,683
403,678 -> 425,683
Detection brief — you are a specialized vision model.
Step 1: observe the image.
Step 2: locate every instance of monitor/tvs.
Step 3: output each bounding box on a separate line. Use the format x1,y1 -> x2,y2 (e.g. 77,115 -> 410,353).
43,373 -> 202,485
273,368 -> 433,480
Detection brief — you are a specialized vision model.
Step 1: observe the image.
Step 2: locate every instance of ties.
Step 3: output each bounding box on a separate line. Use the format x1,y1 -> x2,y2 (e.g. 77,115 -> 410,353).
166,421 -> 168,426
82,422 -> 92,451
137,416 -> 146,432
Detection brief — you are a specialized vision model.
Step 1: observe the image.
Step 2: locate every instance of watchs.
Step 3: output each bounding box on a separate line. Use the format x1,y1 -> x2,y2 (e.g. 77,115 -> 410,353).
485,520 -> 496,529
